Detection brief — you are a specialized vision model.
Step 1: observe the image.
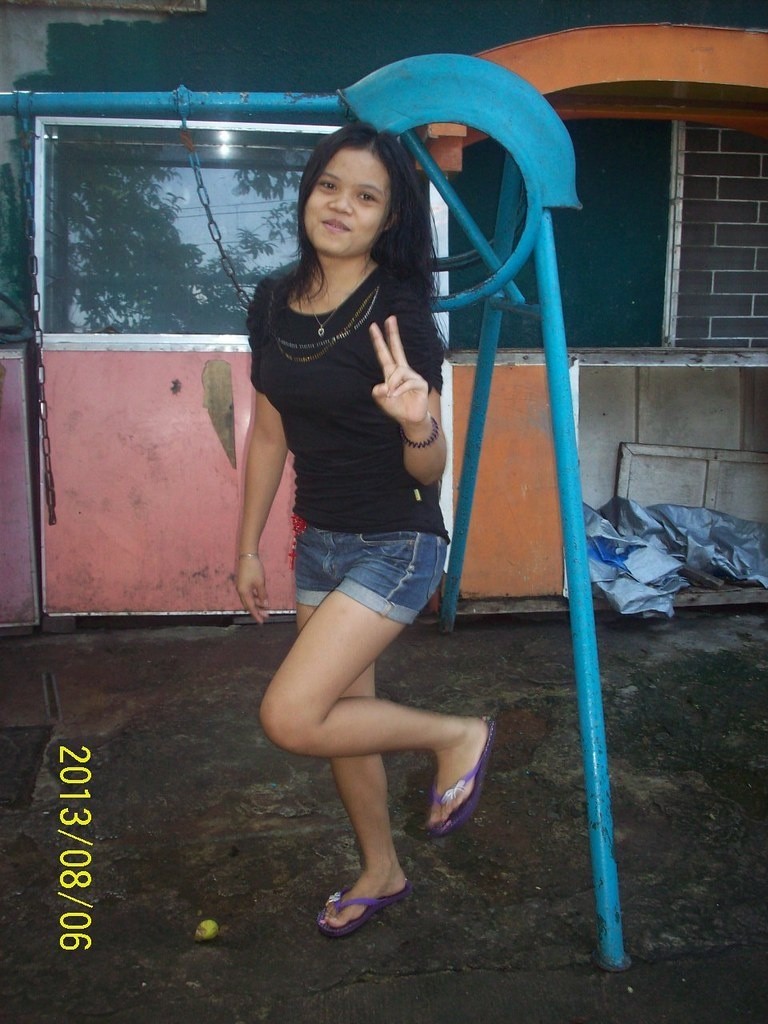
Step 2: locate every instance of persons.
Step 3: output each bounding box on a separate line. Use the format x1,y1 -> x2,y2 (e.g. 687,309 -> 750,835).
233,124 -> 498,938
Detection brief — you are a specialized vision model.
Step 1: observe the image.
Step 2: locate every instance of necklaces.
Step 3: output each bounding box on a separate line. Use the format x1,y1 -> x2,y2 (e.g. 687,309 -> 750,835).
308,268 -> 368,336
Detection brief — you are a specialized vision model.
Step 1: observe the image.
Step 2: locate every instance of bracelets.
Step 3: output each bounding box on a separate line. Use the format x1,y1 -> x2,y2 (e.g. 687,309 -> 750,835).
402,414 -> 440,448
238,553 -> 261,560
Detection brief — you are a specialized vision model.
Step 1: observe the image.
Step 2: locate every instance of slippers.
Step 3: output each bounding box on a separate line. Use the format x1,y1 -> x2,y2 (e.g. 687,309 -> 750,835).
317,880 -> 411,937
428,716 -> 497,835
